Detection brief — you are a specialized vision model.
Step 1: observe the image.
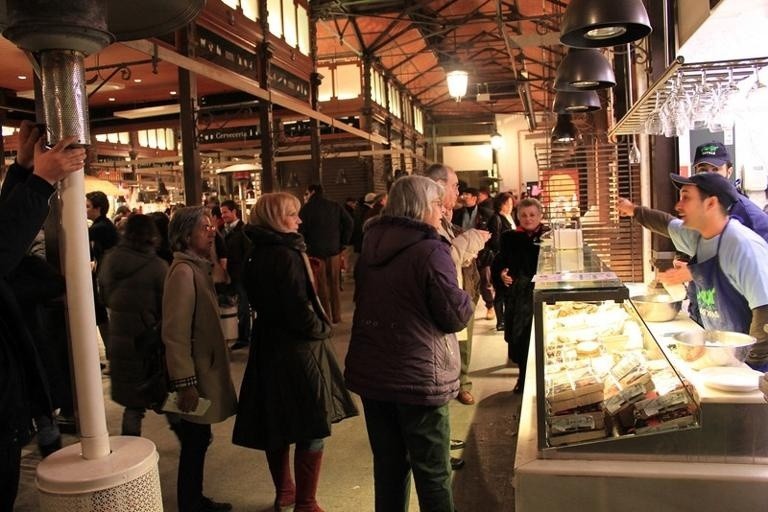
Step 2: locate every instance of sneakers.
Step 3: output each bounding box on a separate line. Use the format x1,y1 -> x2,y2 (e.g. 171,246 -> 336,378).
487,307 -> 495,319
200,495 -> 231,512
231,340 -> 247,350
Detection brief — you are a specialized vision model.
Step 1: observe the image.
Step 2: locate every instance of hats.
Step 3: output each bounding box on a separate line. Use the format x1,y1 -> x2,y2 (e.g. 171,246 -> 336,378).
670,173 -> 739,208
694,142 -> 729,167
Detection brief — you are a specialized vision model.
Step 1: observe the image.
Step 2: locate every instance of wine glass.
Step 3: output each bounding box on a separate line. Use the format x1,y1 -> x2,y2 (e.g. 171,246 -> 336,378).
642,66 -> 766,138
628,130 -> 642,166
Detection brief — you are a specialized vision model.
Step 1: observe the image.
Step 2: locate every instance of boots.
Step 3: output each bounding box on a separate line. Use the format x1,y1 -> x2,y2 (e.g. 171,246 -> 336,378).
264,444 -> 298,511
294,447 -> 325,512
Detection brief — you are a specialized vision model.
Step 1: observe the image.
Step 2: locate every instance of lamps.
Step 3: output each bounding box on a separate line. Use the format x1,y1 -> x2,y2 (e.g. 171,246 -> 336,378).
443,61 -> 470,104
489,125 -> 502,150
548,0 -> 654,145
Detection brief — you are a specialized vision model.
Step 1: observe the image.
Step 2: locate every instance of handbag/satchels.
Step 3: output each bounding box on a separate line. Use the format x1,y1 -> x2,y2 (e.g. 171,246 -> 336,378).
138,318 -> 177,413
478,248 -> 494,267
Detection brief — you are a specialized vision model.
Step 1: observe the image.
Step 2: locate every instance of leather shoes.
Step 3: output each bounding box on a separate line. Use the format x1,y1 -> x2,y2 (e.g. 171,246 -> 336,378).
458,390 -> 474,405
450,457 -> 464,470
451,439 -> 464,450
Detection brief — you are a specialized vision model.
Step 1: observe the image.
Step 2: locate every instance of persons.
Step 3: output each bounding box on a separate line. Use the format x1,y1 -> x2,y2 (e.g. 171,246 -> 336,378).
225,190 -> 361,512
606,169 -> 768,379
656,138 -> 768,327
337,174 -> 476,511
6,156 -> 556,473
159,202 -> 240,512
2,119 -> 90,511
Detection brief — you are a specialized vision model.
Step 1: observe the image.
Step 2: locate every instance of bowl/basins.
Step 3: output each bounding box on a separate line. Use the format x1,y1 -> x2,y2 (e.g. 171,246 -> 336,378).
671,329 -> 759,367
631,294 -> 683,321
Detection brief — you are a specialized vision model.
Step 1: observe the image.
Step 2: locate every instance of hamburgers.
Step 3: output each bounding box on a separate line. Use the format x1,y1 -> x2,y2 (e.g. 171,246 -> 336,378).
576,341 -> 600,359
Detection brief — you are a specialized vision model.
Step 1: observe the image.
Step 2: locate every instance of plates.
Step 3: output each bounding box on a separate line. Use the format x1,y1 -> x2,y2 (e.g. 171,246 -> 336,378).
697,368 -> 764,393
